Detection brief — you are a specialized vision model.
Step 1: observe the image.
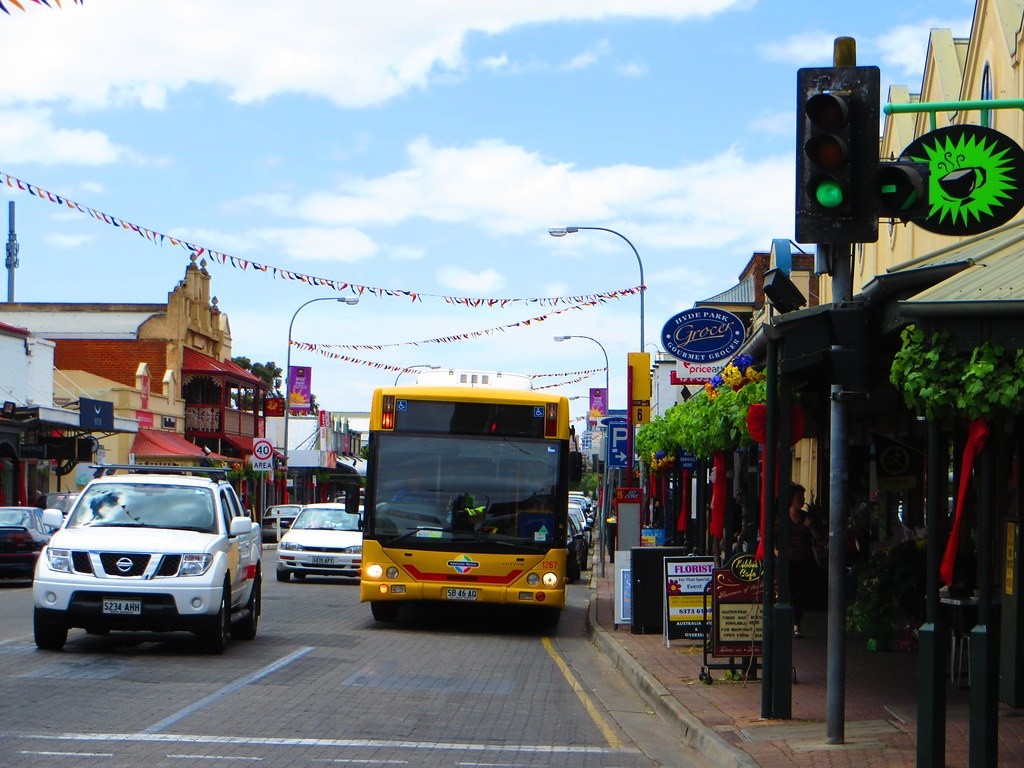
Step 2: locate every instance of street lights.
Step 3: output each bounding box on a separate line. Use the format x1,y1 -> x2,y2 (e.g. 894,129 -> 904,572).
394,364 -> 441,386
548,228 -> 645,351
553,336 -> 608,579
281,297 -> 360,504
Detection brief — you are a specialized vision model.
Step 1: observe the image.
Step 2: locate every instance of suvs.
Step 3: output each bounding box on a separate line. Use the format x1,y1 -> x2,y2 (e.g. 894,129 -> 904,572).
32,462 -> 263,655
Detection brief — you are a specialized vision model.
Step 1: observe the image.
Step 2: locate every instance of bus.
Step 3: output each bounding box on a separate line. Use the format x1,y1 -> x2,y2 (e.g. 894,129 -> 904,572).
346,387 -> 582,629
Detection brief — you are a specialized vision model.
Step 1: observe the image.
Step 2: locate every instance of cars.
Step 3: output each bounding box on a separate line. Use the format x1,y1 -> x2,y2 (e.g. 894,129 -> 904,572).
0,491 -> 82,587
263,503 -> 364,582
566,491 -> 598,583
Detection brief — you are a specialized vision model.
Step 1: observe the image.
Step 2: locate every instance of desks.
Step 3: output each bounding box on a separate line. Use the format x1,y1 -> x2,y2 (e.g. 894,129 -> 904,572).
939,595 -> 980,690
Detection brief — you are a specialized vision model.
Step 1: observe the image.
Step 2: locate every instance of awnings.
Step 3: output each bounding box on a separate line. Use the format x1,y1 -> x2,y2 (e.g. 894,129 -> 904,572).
336,452 -> 367,477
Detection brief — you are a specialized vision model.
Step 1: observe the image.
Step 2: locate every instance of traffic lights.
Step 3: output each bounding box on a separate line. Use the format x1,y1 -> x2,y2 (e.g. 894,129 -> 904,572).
795,66 -> 880,245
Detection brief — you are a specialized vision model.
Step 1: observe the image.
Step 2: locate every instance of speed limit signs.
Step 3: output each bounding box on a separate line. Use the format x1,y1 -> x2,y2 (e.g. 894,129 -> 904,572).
252,439 -> 274,473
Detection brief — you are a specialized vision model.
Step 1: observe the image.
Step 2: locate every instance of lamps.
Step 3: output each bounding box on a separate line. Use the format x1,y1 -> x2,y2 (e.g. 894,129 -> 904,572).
762,267 -> 807,314
679,384 -> 692,402
2,401 -> 16,420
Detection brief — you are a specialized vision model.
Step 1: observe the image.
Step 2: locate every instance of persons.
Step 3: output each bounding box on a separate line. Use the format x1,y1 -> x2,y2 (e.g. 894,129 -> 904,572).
390,463 -> 436,506
771,483 -> 825,639
653,500 -> 662,528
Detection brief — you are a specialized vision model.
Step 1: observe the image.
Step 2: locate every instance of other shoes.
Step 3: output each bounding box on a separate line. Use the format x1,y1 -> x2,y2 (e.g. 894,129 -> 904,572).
793,627 -> 803,639
